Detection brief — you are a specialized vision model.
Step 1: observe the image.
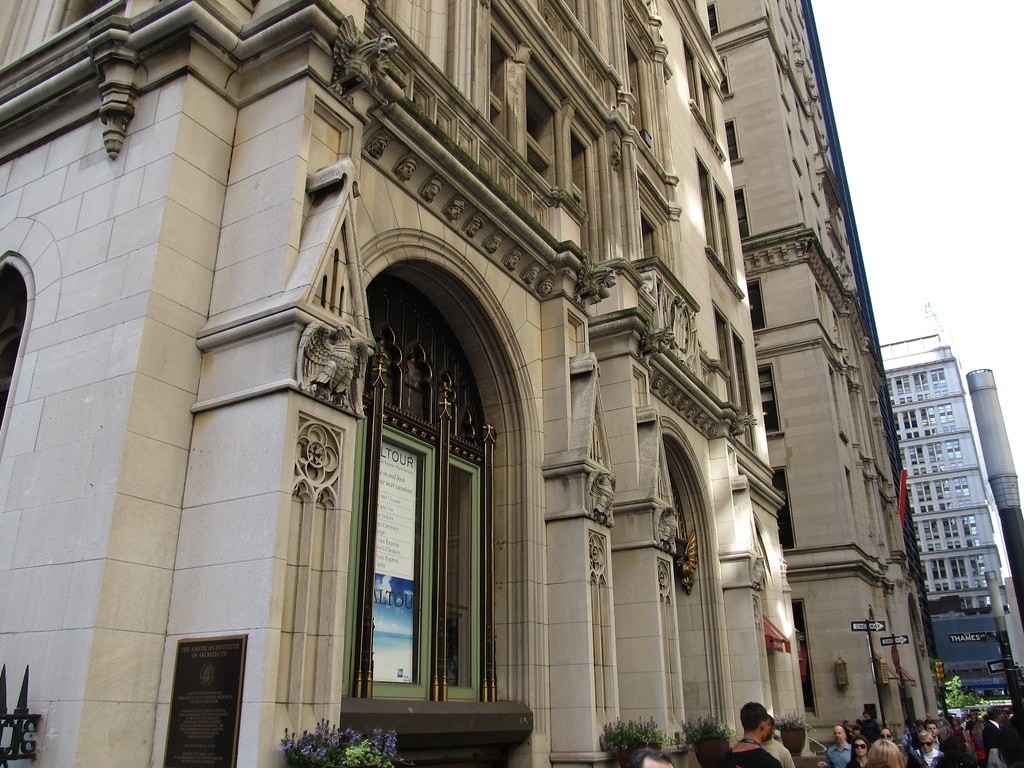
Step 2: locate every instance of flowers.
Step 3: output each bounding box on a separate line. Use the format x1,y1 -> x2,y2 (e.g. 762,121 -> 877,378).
680,713 -> 738,751
603,714 -> 675,751
268,717 -> 413,768
773,710 -> 817,733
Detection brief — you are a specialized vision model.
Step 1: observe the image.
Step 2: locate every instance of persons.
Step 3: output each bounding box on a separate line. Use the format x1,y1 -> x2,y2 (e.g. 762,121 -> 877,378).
712,702 -> 782,768
845,735 -> 870,768
816,725 -> 852,768
842,710 -> 911,768
370,137 -> 556,297
905,707 -> 1024,768
864,739 -> 907,768
628,748 -> 675,768
914,730 -> 944,768
760,714 -> 795,768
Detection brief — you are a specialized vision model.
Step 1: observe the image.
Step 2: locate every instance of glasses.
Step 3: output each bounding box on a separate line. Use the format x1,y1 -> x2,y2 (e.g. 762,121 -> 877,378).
920,742 -> 931,746
880,734 -> 891,738
854,744 -> 866,749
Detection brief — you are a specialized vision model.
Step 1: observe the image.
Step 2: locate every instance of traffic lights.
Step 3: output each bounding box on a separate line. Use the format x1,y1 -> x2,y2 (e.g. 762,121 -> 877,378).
936,662 -> 945,680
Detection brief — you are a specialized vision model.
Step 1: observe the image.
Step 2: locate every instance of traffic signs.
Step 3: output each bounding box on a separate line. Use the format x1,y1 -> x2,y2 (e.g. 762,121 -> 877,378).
851,620 -> 886,632
881,635 -> 909,646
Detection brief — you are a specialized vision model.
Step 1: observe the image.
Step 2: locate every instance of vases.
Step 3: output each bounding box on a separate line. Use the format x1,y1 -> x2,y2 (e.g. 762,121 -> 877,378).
617,749 -> 639,768
780,728 -> 805,757
693,741 -> 729,768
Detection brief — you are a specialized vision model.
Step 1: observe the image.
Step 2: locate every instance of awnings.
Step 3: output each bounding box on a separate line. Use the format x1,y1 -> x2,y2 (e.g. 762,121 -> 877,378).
901,667 -> 916,687
763,614 -> 791,653
874,653 -> 900,681
